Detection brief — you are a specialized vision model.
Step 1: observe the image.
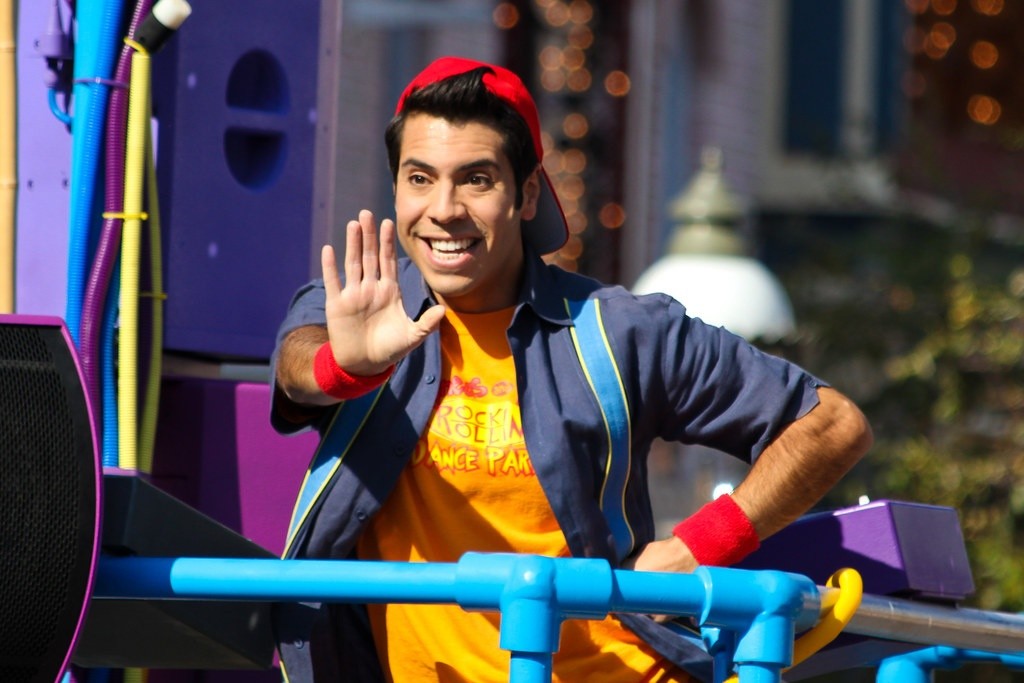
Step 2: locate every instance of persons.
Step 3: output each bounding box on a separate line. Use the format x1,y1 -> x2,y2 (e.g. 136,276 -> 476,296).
267,60 -> 875,683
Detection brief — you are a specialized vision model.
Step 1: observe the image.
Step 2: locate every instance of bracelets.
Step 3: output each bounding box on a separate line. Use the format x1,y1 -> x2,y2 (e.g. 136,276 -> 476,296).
313,341 -> 395,401
676,494 -> 761,570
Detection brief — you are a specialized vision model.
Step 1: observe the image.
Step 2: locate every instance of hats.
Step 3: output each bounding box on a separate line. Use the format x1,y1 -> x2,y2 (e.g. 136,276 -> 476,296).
393,55 -> 571,257
